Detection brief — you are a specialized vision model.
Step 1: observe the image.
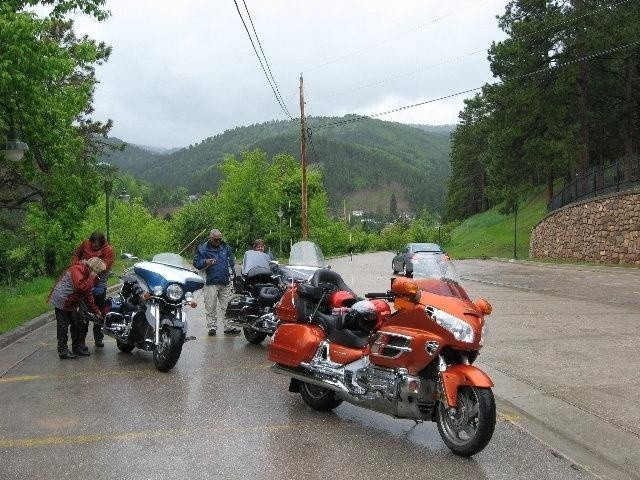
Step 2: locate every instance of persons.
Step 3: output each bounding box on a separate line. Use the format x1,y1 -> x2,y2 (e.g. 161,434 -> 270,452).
47,256 -> 107,360
250,239 -> 275,261
392,252 -> 406,275
193,228 -> 241,336
73,233 -> 114,351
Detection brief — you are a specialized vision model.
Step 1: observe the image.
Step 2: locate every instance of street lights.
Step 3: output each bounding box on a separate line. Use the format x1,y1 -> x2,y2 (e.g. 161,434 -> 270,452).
276,203 -> 284,257
0,101 -> 30,164
104,176 -> 130,243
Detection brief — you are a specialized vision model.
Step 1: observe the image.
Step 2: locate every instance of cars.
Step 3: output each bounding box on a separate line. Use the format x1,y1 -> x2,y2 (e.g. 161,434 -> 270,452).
391,242 -> 449,277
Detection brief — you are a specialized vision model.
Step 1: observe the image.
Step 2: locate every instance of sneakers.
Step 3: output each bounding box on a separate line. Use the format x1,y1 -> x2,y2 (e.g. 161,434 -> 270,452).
224,327 -> 242,335
58,345 -> 91,360
95,339 -> 105,348
208,328 -> 216,336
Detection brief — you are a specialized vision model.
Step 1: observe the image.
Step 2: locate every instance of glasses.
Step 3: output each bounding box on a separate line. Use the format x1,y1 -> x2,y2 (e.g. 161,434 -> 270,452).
211,236 -> 223,242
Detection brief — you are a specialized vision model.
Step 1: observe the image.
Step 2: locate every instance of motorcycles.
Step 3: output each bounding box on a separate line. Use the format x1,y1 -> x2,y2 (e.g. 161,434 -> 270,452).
225,240 -> 331,346
268,250 -> 498,458
100,251 -> 207,372
